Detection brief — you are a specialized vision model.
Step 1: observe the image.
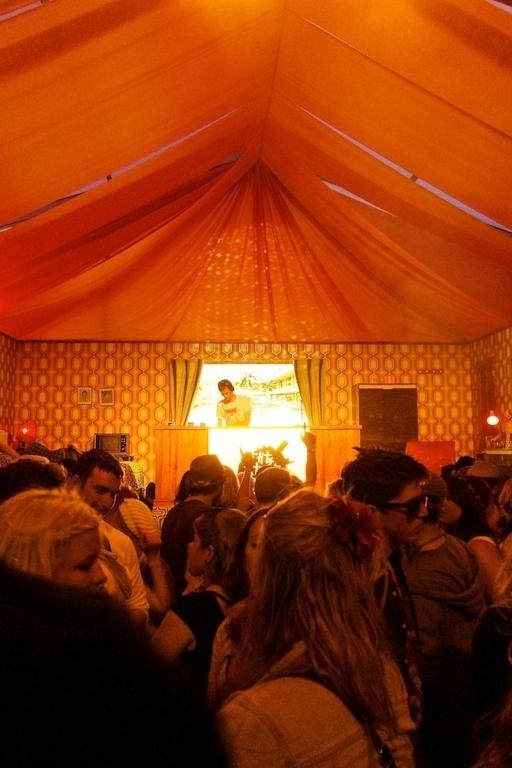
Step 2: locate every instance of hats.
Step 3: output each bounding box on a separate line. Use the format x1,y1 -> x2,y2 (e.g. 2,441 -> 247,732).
183,452 -> 228,490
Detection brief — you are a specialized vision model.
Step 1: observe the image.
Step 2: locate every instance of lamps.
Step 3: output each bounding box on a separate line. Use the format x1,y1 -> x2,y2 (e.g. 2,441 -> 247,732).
486,409 -> 506,451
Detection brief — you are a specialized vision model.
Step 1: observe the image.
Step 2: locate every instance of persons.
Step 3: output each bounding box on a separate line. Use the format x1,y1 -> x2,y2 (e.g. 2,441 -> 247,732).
1,443 -> 512,768
216,380 -> 253,428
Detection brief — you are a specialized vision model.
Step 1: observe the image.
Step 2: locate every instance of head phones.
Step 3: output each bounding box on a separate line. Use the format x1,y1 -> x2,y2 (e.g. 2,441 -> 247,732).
218,380 -> 234,391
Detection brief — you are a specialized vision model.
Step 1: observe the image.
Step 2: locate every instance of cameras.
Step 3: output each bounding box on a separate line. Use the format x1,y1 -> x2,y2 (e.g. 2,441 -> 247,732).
423,493 -> 442,523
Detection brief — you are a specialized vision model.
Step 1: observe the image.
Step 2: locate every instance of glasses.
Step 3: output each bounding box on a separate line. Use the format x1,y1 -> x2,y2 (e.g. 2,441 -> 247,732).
383,491 -> 431,517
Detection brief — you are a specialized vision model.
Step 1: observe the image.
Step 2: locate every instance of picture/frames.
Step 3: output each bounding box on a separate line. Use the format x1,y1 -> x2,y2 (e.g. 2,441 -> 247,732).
77,387 -> 91,404
99,387 -> 114,405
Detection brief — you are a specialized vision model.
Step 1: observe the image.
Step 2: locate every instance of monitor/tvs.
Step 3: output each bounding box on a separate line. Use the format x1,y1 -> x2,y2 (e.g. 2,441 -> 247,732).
93,433 -> 130,457
